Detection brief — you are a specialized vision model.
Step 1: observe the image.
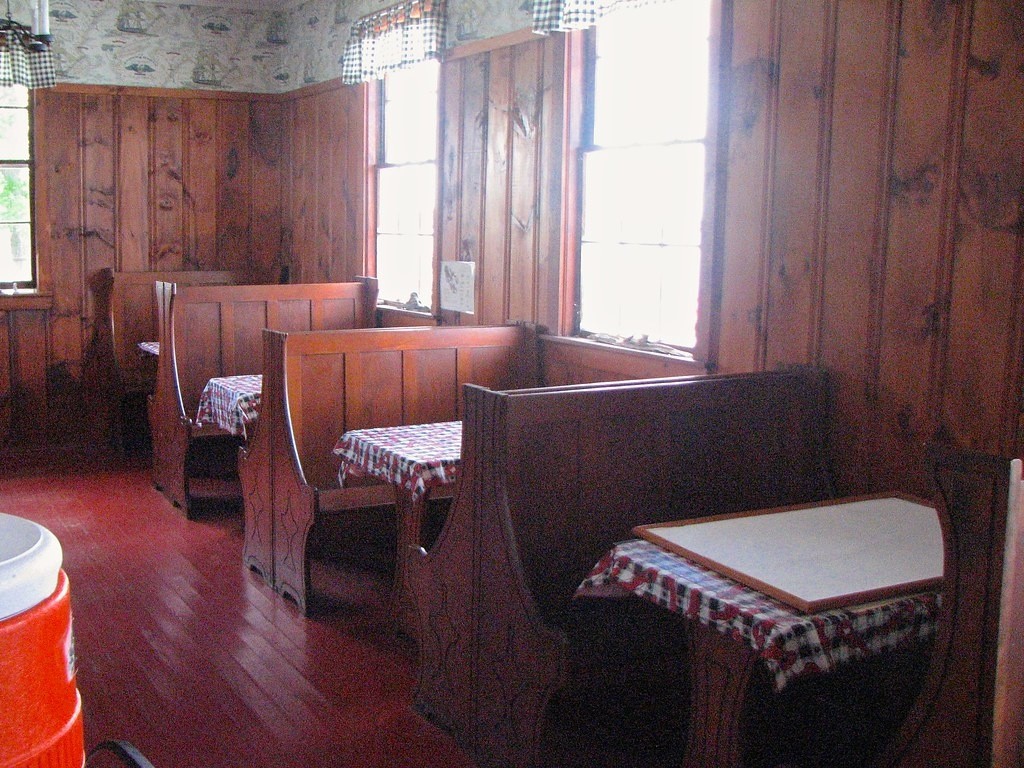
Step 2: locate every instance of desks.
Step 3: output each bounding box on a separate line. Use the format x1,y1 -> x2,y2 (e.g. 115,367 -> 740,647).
570,487 -> 945,768
331,420 -> 462,665
196,374 -> 263,446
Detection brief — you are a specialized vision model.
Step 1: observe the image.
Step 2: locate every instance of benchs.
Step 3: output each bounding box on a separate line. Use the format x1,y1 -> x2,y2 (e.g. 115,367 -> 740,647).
397,361 -> 802,752
146,275 -> 365,499
235,318 -> 524,590
272,324 -> 539,617
80,261 -> 290,457
470,371 -> 848,768
163,276 -> 379,520
775,447 -> 994,768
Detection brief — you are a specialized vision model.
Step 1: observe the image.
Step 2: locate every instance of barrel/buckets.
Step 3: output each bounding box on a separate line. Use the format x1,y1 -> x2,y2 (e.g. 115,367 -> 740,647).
0,512 -> 86,768
